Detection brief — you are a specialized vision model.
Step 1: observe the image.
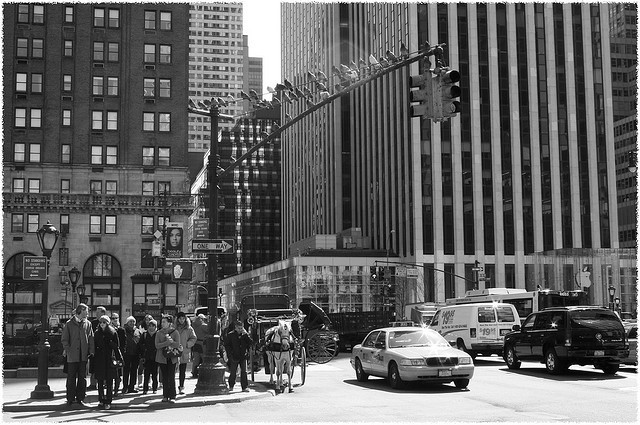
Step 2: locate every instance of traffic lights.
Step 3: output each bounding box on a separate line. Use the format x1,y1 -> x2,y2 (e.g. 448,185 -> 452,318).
172,260 -> 192,281
379,267 -> 384,281
409,72 -> 432,119
433,67 -> 462,124
370,266 -> 377,278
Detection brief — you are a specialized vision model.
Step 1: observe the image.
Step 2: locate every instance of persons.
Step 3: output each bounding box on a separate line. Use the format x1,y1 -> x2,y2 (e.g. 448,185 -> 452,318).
155,315 -> 180,403
61,304 -> 95,408
138,315 -> 154,388
225,322 -> 254,392
141,320 -> 158,394
87,307 -> 109,390
62,309 -> 77,374
169,228 -> 182,250
173,312 -> 197,394
94,316 -> 124,410
110,313 -> 127,397
122,316 -> 141,393
191,314 -> 208,376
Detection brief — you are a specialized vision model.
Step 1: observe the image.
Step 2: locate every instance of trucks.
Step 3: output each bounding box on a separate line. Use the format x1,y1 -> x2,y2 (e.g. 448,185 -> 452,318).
326,312 -> 388,351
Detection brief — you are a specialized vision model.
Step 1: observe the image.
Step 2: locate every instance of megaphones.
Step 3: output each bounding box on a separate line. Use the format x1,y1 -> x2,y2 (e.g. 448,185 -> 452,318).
247,316 -> 255,324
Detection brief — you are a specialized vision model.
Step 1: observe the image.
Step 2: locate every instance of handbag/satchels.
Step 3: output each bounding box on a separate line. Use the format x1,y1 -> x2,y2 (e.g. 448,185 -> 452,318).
111,347 -> 125,367
163,343 -> 183,357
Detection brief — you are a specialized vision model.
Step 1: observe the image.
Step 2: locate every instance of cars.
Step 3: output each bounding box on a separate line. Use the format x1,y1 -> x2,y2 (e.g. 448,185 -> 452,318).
623,323 -> 637,363
350,327 -> 474,389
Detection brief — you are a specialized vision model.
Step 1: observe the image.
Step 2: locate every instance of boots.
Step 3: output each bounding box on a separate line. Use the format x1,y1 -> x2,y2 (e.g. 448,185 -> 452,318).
148,378 -> 151,389
138,375 -> 142,388
178,386 -> 185,394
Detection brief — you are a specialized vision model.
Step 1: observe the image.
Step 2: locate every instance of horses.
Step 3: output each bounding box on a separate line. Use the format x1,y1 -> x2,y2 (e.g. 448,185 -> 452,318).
264,319 -> 295,395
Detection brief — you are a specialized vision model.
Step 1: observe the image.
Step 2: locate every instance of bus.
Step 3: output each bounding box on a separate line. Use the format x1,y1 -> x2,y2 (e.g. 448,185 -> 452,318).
446,288 -> 588,327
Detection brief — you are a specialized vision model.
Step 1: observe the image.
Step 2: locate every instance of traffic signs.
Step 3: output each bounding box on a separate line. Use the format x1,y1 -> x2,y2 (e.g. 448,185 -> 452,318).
192,239 -> 234,255
23,256 -> 47,281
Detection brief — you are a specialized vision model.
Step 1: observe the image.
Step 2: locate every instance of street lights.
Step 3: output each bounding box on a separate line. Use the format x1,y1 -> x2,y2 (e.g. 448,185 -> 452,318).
608,285 -> 616,311
385,230 -> 396,311
615,297 -> 620,311
69,267 -> 81,310
31,220 -> 60,399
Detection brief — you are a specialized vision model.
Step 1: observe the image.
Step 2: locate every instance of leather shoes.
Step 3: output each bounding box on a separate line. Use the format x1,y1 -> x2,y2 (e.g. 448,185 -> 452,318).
98,403 -> 104,409
143,390 -> 147,394
103,385 -> 107,388
153,390 -> 157,394
114,393 -> 120,397
77,401 -> 88,408
87,385 -> 96,390
129,388 -> 138,393
105,404 -> 110,410
67,401 -> 73,405
122,388 -> 127,393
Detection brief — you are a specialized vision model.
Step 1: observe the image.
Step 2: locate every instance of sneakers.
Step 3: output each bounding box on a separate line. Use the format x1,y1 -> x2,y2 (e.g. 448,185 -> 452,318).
163,397 -> 168,402
229,386 -> 233,390
193,374 -> 198,378
243,388 -> 249,392
170,398 -> 175,403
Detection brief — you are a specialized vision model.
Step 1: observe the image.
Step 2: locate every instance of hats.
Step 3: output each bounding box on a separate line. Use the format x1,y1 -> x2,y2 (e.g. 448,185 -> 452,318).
177,312 -> 185,319
126,315 -> 136,323
148,319 -> 157,328
99,315 -> 111,323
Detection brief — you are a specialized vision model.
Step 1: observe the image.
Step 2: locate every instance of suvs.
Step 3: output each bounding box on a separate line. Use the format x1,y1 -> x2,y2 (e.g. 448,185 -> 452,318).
427,303 -> 521,361
504,306 -> 630,375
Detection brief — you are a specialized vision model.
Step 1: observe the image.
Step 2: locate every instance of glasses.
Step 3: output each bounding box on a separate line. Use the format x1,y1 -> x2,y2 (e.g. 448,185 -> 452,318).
129,321 -> 135,322
112,318 -> 118,320
99,322 -> 106,324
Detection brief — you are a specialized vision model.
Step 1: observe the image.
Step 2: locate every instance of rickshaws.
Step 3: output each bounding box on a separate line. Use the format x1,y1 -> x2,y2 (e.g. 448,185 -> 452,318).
299,300 -> 340,364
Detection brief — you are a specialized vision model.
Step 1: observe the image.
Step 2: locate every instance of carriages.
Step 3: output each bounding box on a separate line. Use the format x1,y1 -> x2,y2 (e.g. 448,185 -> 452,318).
239,295 -> 308,395
